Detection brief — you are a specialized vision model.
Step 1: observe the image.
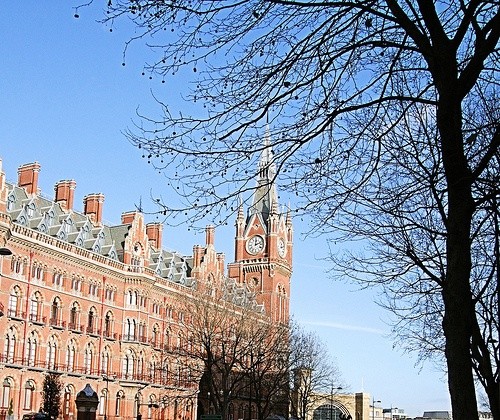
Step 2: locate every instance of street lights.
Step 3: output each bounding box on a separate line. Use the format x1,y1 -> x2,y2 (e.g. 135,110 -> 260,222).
390,407 -> 398,420
372,400 -> 380,420
330,387 -> 343,419
